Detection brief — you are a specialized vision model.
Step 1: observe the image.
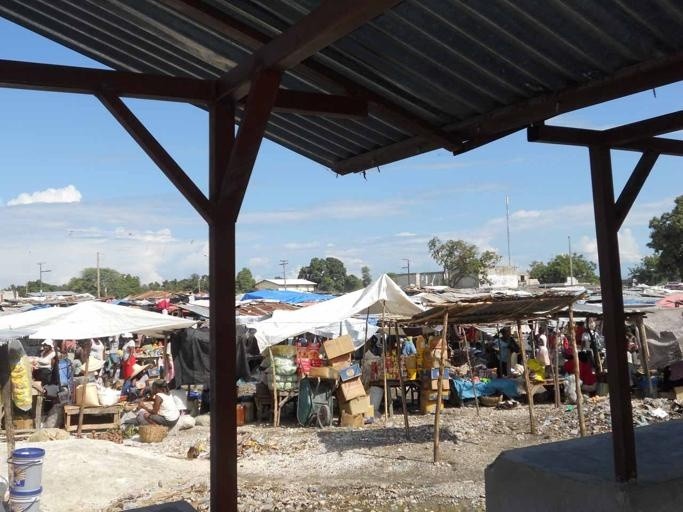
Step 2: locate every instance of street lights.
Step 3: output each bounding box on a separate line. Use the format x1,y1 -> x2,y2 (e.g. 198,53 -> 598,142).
568,233 -> 575,285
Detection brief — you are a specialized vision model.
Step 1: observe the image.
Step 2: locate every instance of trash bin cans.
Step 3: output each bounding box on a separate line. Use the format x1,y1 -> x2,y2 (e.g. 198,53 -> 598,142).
241,396 -> 255,423
596,373 -> 609,397
187,392 -> 200,410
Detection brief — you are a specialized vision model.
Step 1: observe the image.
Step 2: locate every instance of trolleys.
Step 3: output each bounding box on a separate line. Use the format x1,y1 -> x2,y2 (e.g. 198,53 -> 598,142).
295,376 -> 340,427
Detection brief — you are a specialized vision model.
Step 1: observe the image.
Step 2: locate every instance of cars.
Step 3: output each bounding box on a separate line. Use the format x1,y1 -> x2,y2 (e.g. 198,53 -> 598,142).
662,282 -> 682,291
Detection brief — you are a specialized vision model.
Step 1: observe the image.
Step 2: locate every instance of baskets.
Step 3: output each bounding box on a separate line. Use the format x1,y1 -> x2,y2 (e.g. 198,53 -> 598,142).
139,425 -> 167,442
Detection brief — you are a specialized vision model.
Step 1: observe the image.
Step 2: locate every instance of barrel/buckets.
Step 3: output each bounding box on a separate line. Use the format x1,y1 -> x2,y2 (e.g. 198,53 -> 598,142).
7,447 -> 45,492
241,401 -> 255,423
640,376 -> 658,399
237,403 -> 245,426
186,392 -> 200,417
596,382 -> 609,396
367,385 -> 384,411
8,485 -> 43,512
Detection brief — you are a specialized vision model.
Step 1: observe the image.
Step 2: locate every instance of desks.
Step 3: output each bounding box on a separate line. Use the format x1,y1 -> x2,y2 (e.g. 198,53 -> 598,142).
62,402 -> 120,435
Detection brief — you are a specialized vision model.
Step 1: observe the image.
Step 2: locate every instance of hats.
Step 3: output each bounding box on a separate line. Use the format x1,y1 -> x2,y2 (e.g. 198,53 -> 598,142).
80,356 -> 103,372
130,364 -> 152,378
122,333 -> 133,338
41,340 -> 54,348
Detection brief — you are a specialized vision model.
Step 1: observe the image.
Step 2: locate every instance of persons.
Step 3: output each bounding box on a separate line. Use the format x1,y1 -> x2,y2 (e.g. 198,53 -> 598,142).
31,333 -> 180,425
497,322 -> 638,393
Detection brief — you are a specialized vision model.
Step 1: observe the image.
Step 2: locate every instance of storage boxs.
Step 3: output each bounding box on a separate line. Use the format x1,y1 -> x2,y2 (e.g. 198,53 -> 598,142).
322,333 -> 451,428
